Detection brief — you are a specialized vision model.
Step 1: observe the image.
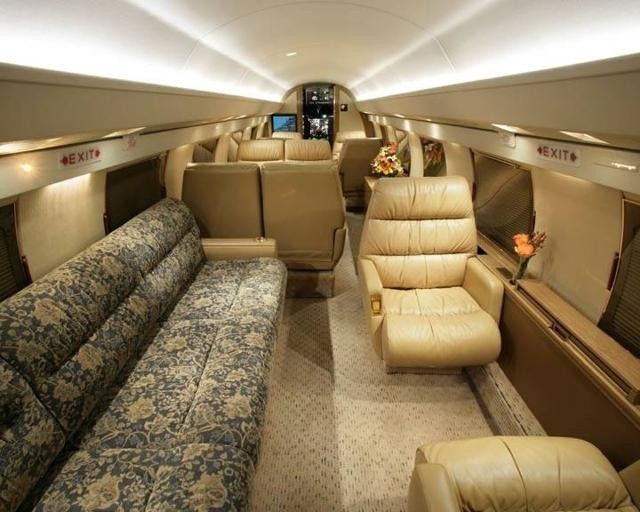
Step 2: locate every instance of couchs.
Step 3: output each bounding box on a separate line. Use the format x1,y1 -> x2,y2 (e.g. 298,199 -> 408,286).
0,200 -> 288,510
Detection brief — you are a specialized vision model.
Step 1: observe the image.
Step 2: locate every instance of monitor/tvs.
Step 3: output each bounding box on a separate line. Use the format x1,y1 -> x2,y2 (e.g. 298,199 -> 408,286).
270,113 -> 298,132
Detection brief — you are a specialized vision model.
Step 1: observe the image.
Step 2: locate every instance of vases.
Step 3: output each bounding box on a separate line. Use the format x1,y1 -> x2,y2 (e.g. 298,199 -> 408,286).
511,256 -> 531,285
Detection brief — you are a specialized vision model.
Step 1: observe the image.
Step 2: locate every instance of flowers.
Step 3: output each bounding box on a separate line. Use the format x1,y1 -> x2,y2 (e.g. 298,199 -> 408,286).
370,142 -> 404,176
512,211 -> 548,256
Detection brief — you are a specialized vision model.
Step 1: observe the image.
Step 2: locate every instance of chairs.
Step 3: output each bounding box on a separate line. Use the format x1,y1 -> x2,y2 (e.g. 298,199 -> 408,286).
407,434 -> 640,511
361,175 -> 506,375
182,137 -> 390,299
